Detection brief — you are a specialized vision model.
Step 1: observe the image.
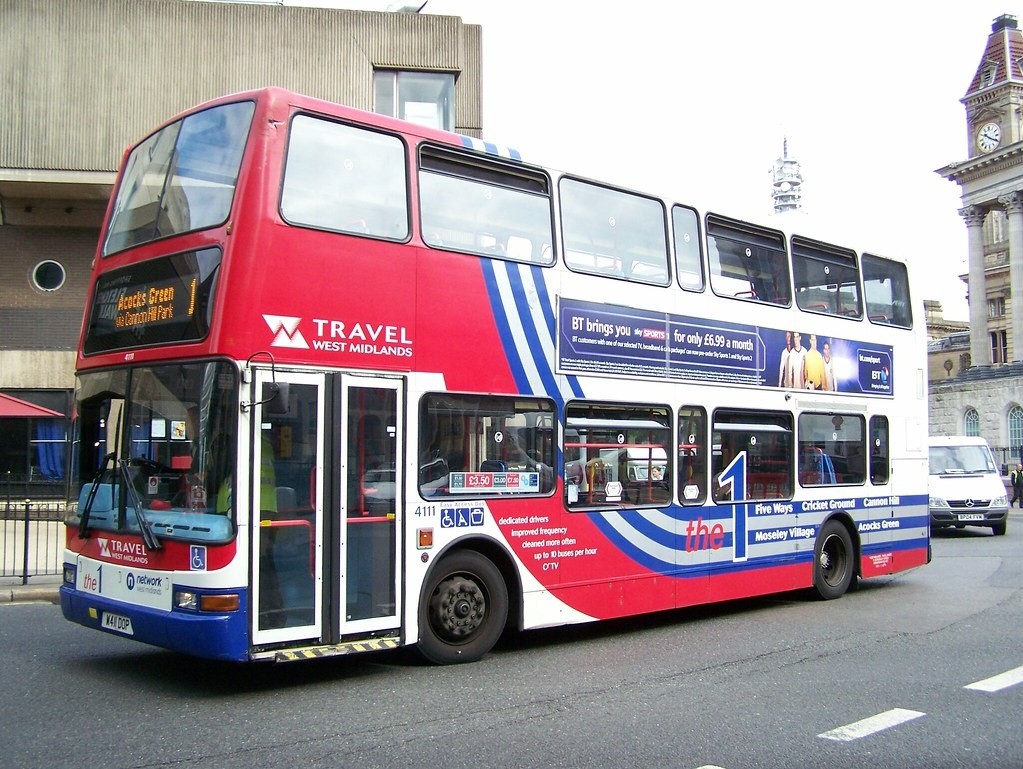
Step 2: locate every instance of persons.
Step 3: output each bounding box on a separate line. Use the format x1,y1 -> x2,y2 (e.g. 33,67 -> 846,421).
1010,463 -> 1023,508
778,331 -> 838,392
213,428 -> 288,632
170,404 -> 234,515
524,449 -> 554,495
650,466 -> 665,481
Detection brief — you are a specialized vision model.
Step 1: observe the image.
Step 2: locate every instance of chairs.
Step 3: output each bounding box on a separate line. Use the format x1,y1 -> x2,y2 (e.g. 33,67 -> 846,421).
481,459 -> 508,473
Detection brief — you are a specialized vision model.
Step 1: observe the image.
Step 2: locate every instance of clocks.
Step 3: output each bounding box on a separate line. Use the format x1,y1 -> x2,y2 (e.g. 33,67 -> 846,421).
977,122 -> 1002,153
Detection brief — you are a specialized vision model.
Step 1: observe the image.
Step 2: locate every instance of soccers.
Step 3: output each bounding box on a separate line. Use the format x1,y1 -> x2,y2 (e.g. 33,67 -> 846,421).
806,380 -> 814,390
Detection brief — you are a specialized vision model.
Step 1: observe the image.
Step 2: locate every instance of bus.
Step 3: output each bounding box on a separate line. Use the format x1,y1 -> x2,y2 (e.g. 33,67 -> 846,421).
61,86 -> 936,666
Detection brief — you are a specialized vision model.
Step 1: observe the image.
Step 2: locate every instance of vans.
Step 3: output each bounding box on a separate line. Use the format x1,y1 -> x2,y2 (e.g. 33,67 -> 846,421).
926,436 -> 1010,534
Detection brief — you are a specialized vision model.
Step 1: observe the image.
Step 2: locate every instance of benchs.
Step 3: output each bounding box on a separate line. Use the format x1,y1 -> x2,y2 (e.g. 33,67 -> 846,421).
590,480 -> 668,505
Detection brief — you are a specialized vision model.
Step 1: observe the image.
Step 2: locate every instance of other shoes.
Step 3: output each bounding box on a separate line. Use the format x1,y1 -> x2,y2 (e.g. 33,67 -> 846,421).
1010,501 -> 1023,508
274,606 -> 288,628
259,611 -> 270,630
